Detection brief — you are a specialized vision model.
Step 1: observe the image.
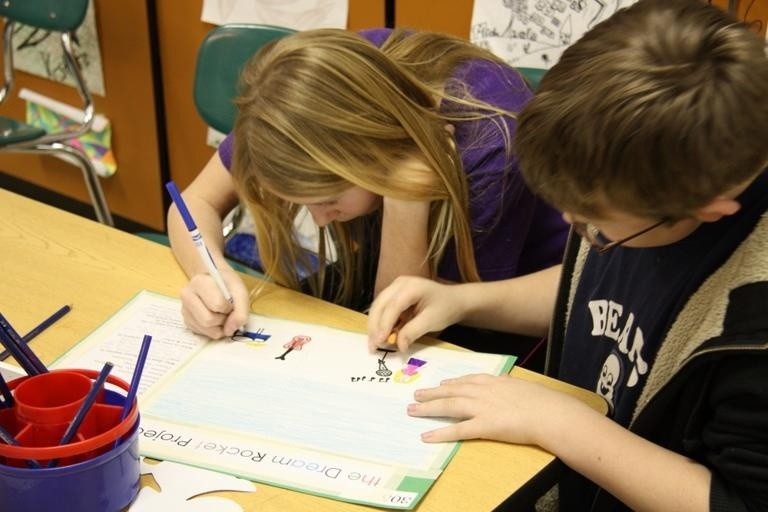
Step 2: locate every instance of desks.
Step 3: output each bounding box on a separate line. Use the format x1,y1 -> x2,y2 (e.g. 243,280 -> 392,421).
0,185 -> 613,512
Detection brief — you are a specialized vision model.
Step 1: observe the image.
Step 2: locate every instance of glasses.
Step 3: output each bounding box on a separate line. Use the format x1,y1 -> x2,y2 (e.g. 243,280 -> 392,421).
564,215 -> 674,257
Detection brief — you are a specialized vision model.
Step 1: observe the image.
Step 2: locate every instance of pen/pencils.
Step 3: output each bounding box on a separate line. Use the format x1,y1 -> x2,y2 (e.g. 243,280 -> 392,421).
114,334 -> 152,448
47,333 -> 49,335
0,427 -> 40,469
0,325 -> 41,377
0,372 -> 16,408
0,302 -> 75,361
383,317 -> 406,350
0,313 -> 49,373
44,360 -> 114,468
165,181 -> 248,336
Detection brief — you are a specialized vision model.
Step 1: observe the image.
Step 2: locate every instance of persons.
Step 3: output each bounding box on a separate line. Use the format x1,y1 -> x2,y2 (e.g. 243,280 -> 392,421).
168,28 -> 570,373
367,1 -> 767,512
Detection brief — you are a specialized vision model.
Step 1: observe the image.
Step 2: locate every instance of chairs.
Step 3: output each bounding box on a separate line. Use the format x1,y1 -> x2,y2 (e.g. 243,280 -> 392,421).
135,22 -> 302,281
0,0 -> 116,229
